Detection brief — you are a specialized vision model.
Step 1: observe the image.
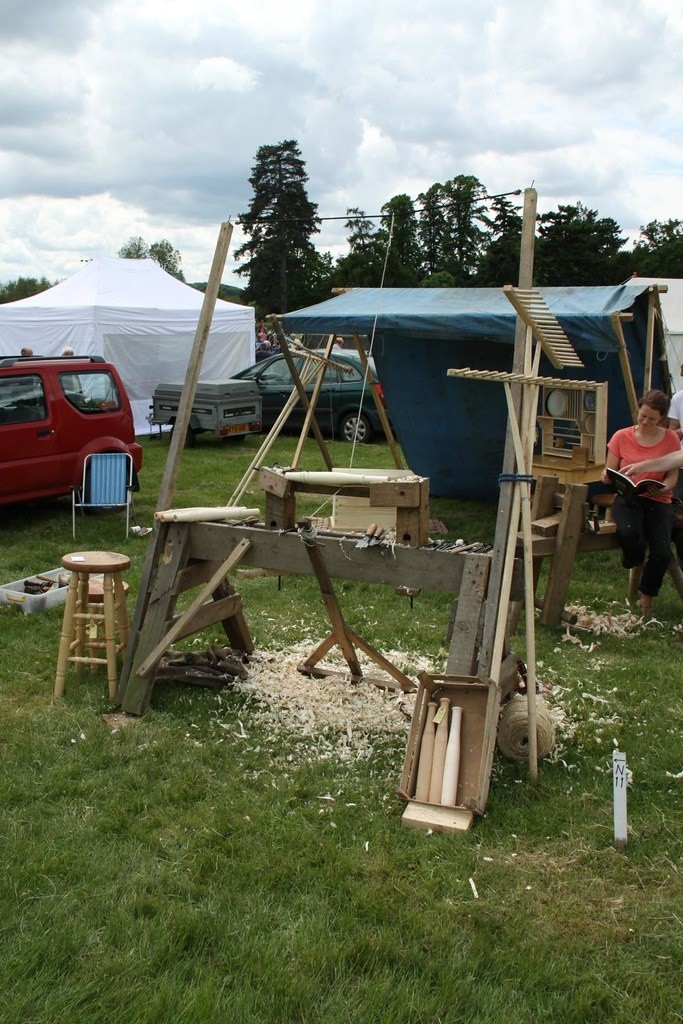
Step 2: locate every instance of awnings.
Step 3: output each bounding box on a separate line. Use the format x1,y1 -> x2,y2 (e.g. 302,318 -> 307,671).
268,286 -> 673,503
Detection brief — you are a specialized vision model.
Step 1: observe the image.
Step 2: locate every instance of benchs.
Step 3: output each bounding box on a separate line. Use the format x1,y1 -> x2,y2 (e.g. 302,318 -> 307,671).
510,522 -> 682,637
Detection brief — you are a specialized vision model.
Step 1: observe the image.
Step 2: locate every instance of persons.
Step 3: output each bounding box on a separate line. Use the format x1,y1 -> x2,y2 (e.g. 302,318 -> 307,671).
255,321 -> 304,362
253,361 -> 290,385
666,389 -> 683,571
601,390 -> 680,621
333,338 -> 344,349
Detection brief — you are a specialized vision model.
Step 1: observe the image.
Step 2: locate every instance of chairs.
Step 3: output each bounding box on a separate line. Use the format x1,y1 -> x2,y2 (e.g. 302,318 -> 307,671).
70,452 -> 138,544
4,389 -> 40,424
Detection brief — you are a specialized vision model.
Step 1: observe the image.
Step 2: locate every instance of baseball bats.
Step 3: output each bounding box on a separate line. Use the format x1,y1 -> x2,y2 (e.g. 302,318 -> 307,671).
152,505 -> 261,524
284,469 -> 390,486
413,695 -> 464,806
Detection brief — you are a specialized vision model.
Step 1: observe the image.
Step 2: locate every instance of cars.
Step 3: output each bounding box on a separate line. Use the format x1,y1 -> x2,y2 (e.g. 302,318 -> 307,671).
228,349 -> 393,444
0,355 -> 142,517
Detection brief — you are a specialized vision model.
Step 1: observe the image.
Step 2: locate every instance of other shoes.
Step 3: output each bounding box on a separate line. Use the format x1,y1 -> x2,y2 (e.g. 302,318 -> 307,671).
629,562 -> 642,595
636,600 -> 654,623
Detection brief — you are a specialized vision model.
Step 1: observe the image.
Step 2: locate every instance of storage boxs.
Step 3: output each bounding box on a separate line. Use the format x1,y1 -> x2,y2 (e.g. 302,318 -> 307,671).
392,670 -> 503,816
0,566 -> 105,615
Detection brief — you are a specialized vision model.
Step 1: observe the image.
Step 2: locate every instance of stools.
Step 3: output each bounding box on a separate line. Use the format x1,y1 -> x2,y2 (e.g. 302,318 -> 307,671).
53,551 -> 132,702
74,579 -> 130,677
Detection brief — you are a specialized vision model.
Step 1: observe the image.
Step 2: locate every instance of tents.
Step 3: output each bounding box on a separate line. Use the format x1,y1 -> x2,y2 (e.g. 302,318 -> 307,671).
0,258 -> 255,435
624,272 -> 683,391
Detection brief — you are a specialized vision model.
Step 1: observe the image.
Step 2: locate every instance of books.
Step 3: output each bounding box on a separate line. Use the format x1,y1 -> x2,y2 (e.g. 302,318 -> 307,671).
605,468 -> 665,505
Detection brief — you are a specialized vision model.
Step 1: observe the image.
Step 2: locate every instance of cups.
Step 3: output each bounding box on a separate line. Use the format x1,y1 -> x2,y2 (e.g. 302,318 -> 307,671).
130,526 -> 141,533
